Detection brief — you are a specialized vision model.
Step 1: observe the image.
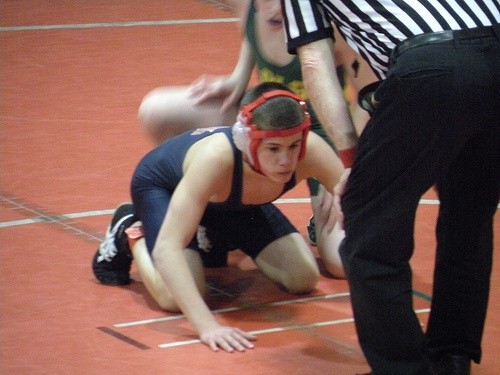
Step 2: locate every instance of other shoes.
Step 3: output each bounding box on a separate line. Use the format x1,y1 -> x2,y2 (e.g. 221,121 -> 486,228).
307,214 -> 316,246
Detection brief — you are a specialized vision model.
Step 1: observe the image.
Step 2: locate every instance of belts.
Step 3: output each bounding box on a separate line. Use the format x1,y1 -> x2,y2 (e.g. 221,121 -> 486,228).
387,25 -> 499,56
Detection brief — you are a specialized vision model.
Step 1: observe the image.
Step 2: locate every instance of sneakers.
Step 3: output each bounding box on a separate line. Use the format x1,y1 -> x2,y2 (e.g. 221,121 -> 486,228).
192,225 -> 225,269
91,203 -> 140,285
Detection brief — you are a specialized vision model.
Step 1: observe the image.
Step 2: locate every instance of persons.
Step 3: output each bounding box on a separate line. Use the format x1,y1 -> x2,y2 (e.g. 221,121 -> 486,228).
280,0 -> 499,374
93,83 -> 346,352
137,0 -> 379,283
